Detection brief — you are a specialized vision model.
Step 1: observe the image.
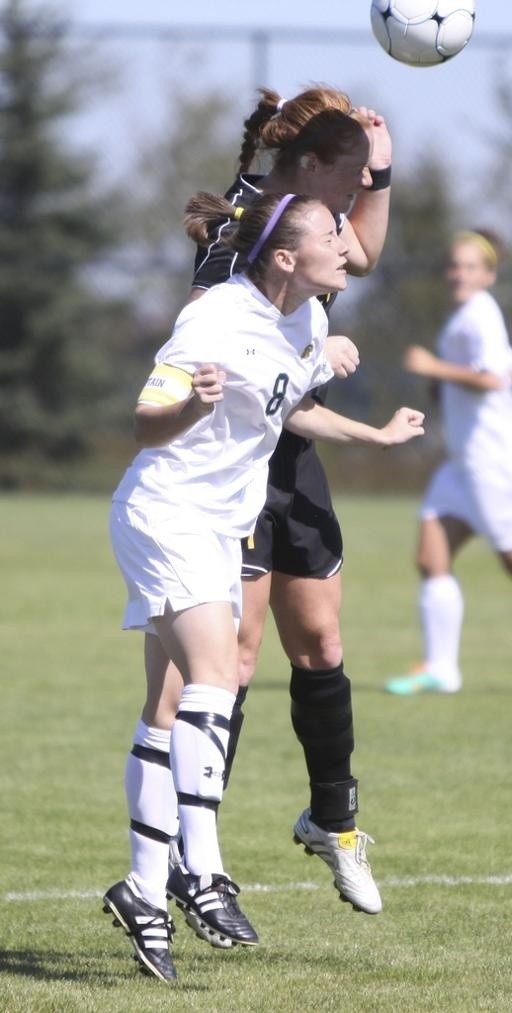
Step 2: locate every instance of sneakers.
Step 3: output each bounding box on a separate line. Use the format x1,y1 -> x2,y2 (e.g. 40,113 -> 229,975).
292,808 -> 384,916
166,833 -> 260,950
102,879 -> 178,983
383,662 -> 463,697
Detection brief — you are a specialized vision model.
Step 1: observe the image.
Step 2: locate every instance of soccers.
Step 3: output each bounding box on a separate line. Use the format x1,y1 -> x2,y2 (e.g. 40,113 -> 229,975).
371,1 -> 474,66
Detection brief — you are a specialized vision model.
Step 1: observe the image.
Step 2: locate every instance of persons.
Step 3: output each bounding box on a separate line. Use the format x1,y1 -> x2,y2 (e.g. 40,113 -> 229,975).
166,83 -> 394,950
380,225 -> 512,695
99,191 -> 427,987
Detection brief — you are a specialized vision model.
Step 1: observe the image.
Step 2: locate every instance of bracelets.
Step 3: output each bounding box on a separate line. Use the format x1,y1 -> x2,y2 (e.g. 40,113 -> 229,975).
365,165 -> 391,192
